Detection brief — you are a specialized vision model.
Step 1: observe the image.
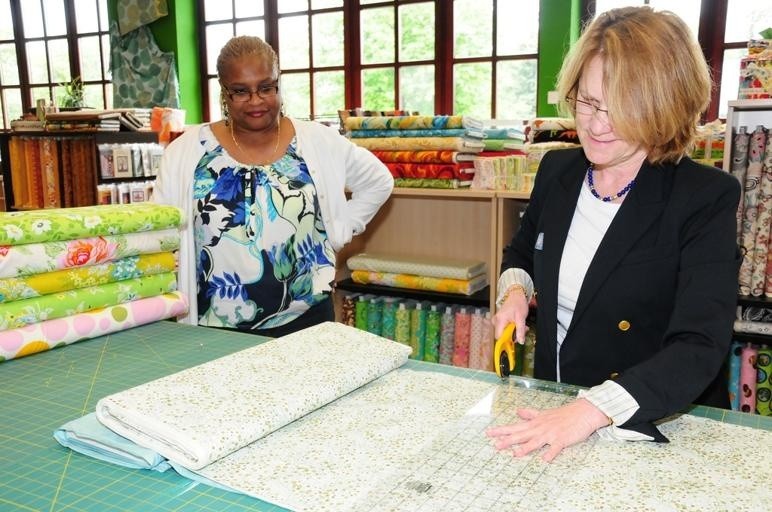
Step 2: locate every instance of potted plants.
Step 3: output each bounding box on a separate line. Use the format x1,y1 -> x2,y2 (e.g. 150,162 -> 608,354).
54,75 -> 95,126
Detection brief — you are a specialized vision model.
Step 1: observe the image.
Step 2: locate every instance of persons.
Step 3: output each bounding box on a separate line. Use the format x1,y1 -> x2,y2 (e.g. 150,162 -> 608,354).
486,7 -> 744,465
150,36 -> 396,342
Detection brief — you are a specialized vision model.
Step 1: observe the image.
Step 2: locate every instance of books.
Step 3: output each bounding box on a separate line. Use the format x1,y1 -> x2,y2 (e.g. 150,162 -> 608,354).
10,97 -> 155,133
96,140 -> 164,205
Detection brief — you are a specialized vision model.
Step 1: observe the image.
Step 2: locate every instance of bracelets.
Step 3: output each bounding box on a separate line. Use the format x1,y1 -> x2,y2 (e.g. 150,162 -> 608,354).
498,285 -> 530,306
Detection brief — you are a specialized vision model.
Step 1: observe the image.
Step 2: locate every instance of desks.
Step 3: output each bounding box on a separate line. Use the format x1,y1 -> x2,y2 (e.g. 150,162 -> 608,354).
1,320 -> 772,511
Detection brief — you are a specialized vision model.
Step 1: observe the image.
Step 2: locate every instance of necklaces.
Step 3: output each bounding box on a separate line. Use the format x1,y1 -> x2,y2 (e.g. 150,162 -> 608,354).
226,118 -> 283,163
589,162 -> 645,202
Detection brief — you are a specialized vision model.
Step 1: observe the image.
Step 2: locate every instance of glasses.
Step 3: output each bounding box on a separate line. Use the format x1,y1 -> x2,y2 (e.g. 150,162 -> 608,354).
565,79 -> 608,117
220,77 -> 279,102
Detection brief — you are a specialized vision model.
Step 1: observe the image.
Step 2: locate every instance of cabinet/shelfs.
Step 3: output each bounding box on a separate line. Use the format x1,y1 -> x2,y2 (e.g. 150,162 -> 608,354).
5,127 -> 183,210
495,191 -> 537,375
335,187 -> 500,372
721,102 -> 772,416
